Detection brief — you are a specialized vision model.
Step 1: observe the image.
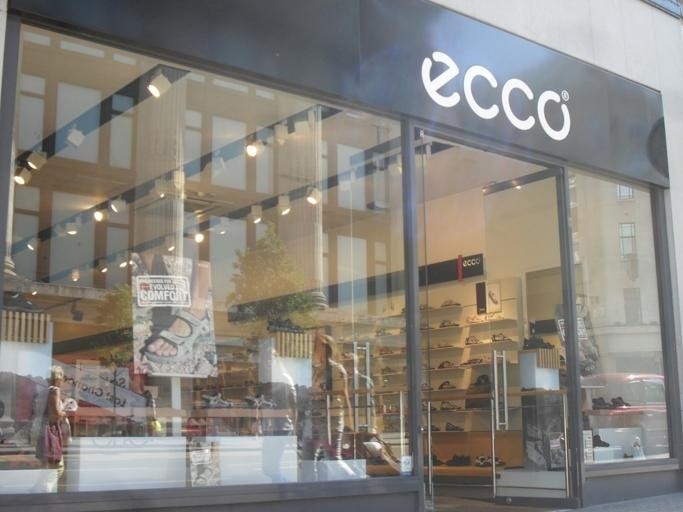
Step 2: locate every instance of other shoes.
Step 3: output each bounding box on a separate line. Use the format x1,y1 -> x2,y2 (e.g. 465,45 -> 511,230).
181,298 -> 514,467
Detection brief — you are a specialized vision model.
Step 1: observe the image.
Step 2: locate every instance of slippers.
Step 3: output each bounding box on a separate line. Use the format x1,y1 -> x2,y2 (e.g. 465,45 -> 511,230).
523,337 -> 555,350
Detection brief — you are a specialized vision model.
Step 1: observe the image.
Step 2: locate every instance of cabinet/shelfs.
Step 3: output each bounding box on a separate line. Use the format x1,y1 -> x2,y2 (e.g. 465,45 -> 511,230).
194,303 -> 519,476
519,389 -> 568,470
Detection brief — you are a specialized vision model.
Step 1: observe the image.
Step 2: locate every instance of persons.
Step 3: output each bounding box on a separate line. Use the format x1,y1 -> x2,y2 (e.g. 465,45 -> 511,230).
574,291 -> 611,448
138,252 -> 211,365
342,350 -> 378,478
258,346 -> 298,483
124,367 -> 157,436
34,365 -> 74,483
299,333 -> 358,480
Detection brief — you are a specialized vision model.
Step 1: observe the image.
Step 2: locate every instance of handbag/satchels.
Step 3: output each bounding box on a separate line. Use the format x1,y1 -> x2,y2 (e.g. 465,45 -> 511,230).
35,417 -> 62,464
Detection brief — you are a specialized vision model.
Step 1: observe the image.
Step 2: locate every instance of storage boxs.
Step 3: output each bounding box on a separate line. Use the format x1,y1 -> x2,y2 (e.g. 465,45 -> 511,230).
518,348 -> 560,391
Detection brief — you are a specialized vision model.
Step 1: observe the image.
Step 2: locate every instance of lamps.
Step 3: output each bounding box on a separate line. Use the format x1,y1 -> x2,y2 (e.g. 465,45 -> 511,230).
93,198 -> 126,222
194,231 -> 204,243
251,205 -> 262,225
25,237 -> 39,252
306,187 -> 322,205
165,235 -> 175,251
67,129 -> 84,150
72,268 -> 80,282
218,216 -> 229,235
14,149 -> 48,186
245,122 -> 309,158
98,259 -> 109,273
65,221 -> 78,236
278,196 -> 291,215
154,177 -> 169,198
119,252 -> 140,270
147,74 -> 171,98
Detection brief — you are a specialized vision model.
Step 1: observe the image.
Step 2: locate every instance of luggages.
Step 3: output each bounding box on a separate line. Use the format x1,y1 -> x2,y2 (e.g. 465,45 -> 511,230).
344,426 -> 402,477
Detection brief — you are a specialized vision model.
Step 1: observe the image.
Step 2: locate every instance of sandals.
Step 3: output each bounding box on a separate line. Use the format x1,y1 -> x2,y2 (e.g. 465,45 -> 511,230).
143,308 -> 210,363
591,396 -> 631,410
593,435 -> 610,448
